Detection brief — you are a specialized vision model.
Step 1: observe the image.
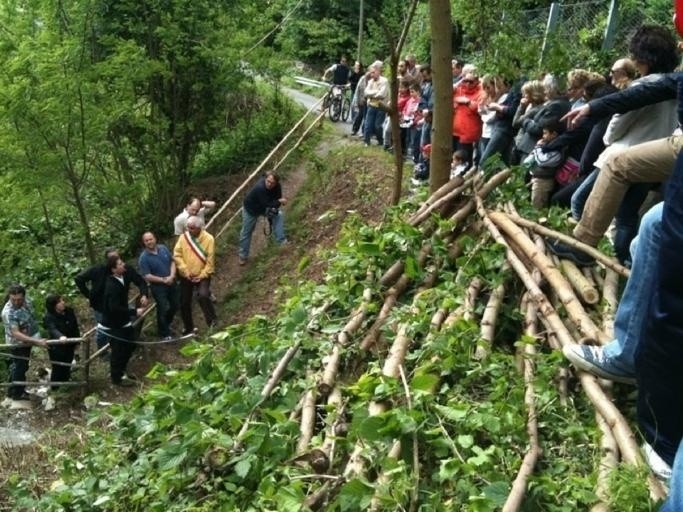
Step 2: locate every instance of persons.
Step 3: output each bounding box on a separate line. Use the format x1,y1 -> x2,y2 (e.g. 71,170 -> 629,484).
238,170 -> 287,264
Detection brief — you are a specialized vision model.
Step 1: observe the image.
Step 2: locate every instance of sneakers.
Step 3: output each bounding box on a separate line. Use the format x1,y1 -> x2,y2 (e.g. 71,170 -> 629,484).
6,323 -> 224,402
351,128 -> 421,167
563,340 -> 639,386
544,234 -> 598,266
237,253 -> 249,265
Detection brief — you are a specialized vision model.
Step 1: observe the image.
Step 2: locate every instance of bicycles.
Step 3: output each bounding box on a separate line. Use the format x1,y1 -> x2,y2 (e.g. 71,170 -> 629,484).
320,77 -> 353,124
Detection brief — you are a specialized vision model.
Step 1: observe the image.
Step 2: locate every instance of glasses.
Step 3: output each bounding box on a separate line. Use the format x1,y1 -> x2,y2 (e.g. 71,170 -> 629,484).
608,69 -> 619,73
462,79 -> 475,85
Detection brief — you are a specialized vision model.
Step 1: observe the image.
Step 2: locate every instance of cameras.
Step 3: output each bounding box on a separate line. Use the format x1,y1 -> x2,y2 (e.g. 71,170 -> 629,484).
265,207 -> 282,217
